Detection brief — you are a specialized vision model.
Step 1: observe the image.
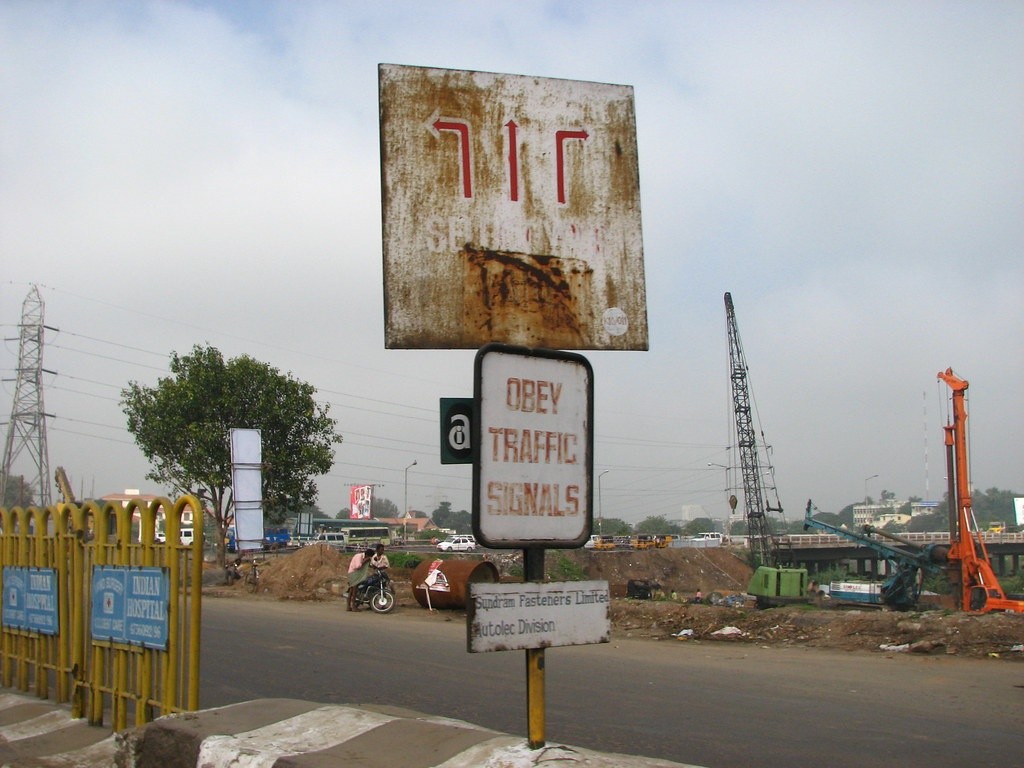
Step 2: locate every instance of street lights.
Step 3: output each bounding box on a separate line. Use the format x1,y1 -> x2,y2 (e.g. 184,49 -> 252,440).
865,474 -> 879,522
404,461 -> 417,545
599,470 -> 609,540
707,463 -> 730,545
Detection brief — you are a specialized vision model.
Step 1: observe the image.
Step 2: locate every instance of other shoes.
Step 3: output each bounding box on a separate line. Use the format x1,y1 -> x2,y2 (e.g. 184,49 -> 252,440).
352,606 -> 360,612
345,606 -> 351,611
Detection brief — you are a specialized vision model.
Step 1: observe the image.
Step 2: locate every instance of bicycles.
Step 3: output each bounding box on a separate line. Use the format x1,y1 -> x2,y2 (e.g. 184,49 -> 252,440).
245,556 -> 260,593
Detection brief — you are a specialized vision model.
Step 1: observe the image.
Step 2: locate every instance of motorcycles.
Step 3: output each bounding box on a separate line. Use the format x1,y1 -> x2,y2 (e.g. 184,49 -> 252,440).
341,556 -> 396,614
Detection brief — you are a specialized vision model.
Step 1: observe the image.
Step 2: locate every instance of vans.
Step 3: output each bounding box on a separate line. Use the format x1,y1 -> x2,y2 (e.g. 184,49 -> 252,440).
583,535 -> 600,550
180,529 -> 193,545
302,533 -> 346,552
691,533 -> 722,548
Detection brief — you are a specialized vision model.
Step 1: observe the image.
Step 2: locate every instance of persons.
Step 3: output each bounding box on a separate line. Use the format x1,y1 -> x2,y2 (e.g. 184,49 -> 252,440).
696,589 -> 702,599
431,536 -> 436,546
344,548 -> 374,612
369,544 -> 390,571
671,589 -> 677,600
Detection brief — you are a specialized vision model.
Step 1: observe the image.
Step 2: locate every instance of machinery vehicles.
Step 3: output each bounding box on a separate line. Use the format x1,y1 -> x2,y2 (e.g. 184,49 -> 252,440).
55,466 -> 116,541
803,367 -> 1024,615
724,291 -> 815,610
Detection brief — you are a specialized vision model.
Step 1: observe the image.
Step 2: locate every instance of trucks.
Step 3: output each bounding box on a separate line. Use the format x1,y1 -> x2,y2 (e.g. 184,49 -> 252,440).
225,525 -> 293,554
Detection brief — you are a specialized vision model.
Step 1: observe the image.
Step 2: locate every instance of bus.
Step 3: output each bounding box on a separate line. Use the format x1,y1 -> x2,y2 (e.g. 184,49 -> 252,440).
340,526 -> 390,547
293,519 -> 390,540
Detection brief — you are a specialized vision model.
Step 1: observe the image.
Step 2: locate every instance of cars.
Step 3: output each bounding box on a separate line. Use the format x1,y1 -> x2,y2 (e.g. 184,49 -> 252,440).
436,534 -> 476,553
